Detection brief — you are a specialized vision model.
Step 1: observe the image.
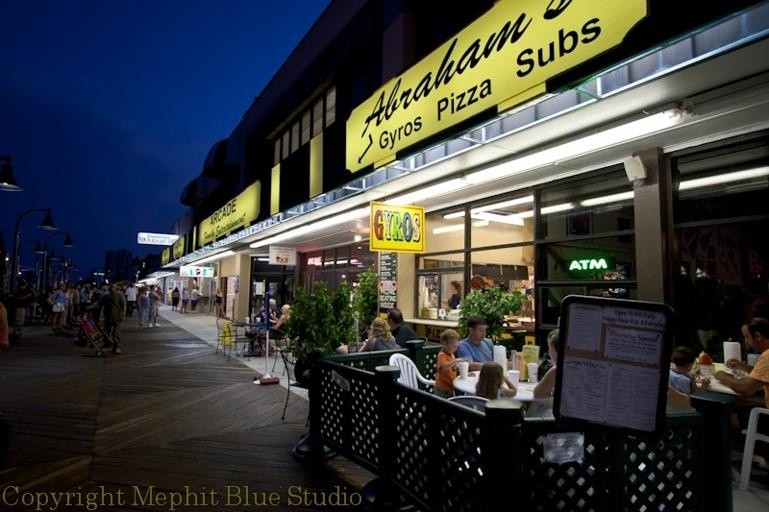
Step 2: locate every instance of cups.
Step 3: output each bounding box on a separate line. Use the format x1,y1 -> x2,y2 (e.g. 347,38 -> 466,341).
526,363 -> 540,383
508,370 -> 520,389
256,317 -> 260,323
458,363 -> 470,379
245,317 -> 250,324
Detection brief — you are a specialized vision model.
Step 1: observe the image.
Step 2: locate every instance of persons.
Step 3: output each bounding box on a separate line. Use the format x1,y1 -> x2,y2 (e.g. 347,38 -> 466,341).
386,310 -> 417,348
434,317 -> 560,410
0,278 -> 162,357
165,284 -> 202,313
716,317 -> 769,478
296,342 -> 349,385
245,298 -> 282,354
425,273 -> 462,308
669,346 -> 709,397
358,317 -> 402,353
215,288 -> 224,320
256,304 -> 292,355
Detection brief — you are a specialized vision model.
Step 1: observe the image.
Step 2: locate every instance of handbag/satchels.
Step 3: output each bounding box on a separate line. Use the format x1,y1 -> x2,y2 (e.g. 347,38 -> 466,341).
537,360 -> 554,382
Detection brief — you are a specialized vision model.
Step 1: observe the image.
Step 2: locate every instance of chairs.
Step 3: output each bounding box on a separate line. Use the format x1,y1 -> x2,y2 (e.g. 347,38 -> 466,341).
388,353 -> 441,393
446,395 -> 492,414
735,398 -> 769,494
214,315 -> 429,427
525,398 -> 555,421
665,386 -> 693,413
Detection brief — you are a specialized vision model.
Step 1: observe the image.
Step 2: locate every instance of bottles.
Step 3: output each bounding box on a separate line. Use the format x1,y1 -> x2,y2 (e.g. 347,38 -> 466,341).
259,377 -> 280,385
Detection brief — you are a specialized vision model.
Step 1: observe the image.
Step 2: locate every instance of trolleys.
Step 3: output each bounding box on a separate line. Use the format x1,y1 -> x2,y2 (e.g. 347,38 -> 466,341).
70,305 -> 121,358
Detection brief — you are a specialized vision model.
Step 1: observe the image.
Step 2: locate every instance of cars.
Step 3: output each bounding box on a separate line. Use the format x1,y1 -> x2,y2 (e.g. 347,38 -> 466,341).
2,264 -> 58,326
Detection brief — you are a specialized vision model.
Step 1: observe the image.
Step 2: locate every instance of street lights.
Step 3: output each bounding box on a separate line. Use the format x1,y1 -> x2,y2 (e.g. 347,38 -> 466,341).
135,270 -> 141,283
16,239 -> 49,292
0,153 -> 24,192
46,257 -> 78,290
9,209 -> 60,296
142,261 -> 148,279
40,229 -> 76,294
